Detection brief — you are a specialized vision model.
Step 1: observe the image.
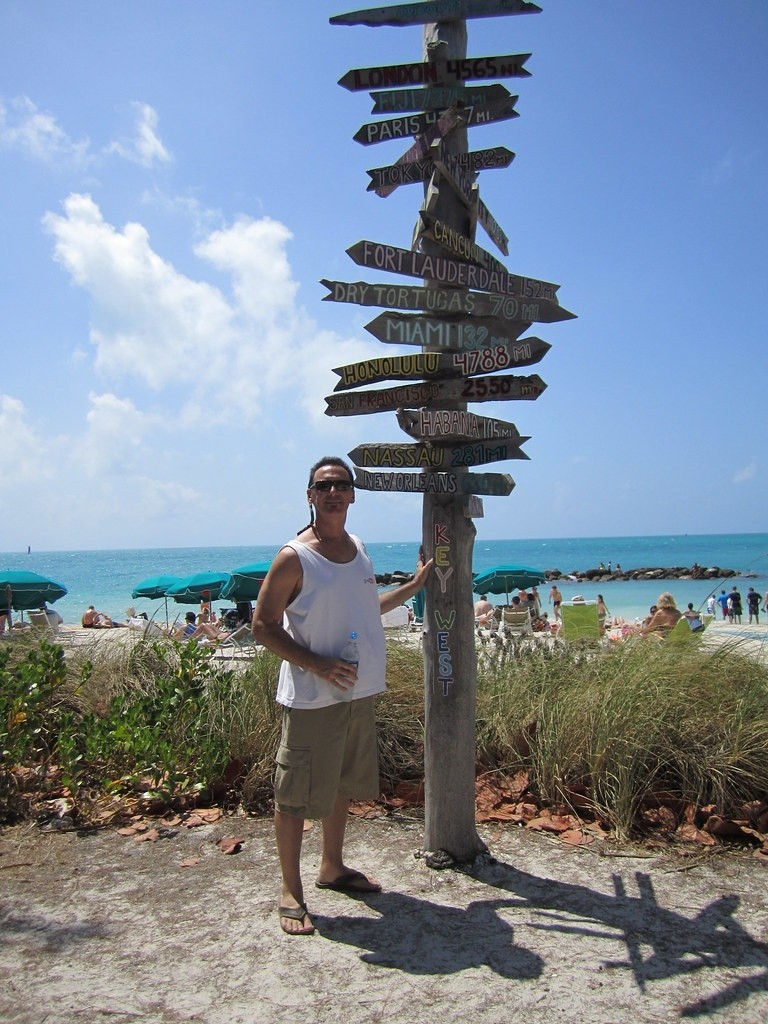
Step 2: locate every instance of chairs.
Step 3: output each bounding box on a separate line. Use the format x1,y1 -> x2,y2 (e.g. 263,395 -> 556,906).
27,601 -> 259,660
476,600 -> 715,648
408,586 -> 424,631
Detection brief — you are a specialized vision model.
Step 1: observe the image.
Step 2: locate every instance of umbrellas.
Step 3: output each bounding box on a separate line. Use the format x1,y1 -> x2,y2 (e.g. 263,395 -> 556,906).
163,570 -> 230,622
413,586 -> 425,622
472,561 -> 546,606
131,572 -> 183,627
218,559 -> 275,602
0,569 -> 68,630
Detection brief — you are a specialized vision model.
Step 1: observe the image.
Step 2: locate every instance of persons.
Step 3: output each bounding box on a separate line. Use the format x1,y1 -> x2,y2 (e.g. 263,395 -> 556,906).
600,561 -> 620,574
40,604 -> 63,627
612,616 -> 630,629
692,563 -> 699,571
548,586 -> 563,621
0,591 -> 13,632
644,592 -> 697,633
707,585 -> 768,625
172,600 -> 253,643
598,594 -> 610,615
475,586 -> 548,629
82,605 -> 128,628
250,457 -> 434,936
572,595 -> 586,606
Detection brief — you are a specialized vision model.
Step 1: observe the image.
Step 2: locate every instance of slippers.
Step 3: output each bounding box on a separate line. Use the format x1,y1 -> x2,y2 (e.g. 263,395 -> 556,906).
315,871 -> 382,892
279,902 -> 316,935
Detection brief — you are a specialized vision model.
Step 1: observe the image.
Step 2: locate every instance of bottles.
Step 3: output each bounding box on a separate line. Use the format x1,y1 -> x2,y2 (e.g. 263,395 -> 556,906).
330,631 -> 360,703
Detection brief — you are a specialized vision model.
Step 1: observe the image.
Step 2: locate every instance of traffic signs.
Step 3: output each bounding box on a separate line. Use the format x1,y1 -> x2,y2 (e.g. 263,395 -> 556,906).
430,138 -> 510,256
329,0 -> 543,27
375,100 -> 463,198
346,436 -> 532,467
337,53 -> 532,92
418,210 -> 509,274
395,407 -> 520,442
352,467 -> 516,496
330,337 -> 552,392
411,168 -> 440,253
353,95 -> 520,146
364,311 -> 533,351
319,279 -> 578,323
345,240 -> 561,305
369,84 -> 511,115
366,146 -> 516,193
324,374 -> 548,417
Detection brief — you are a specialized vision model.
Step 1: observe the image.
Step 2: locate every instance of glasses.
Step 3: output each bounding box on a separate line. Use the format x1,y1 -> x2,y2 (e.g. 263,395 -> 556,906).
309,480 -> 354,491
